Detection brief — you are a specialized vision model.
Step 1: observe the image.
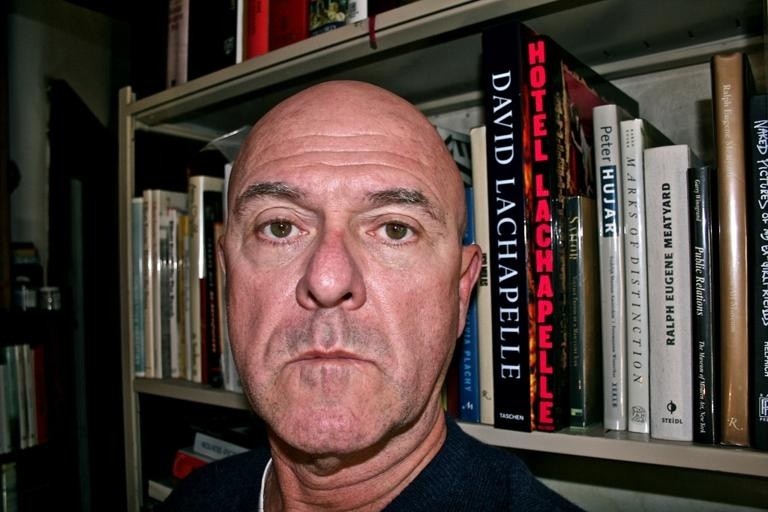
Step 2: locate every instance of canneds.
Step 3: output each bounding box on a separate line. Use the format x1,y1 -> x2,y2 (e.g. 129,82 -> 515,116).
39,287 -> 61,310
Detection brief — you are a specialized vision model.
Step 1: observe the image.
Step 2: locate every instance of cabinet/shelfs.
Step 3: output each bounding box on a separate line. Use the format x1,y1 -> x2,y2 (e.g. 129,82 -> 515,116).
118,0 -> 768,512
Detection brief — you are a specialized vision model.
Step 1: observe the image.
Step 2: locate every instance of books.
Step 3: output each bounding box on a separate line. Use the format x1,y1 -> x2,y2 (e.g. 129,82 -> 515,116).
432,15 -> 768,451
130,161 -> 269,511
0,342 -> 47,456
1,462 -> 18,512
128,2 -> 411,100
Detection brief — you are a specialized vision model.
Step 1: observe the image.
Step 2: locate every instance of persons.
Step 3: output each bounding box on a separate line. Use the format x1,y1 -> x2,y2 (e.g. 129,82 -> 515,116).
150,79 -> 581,511
